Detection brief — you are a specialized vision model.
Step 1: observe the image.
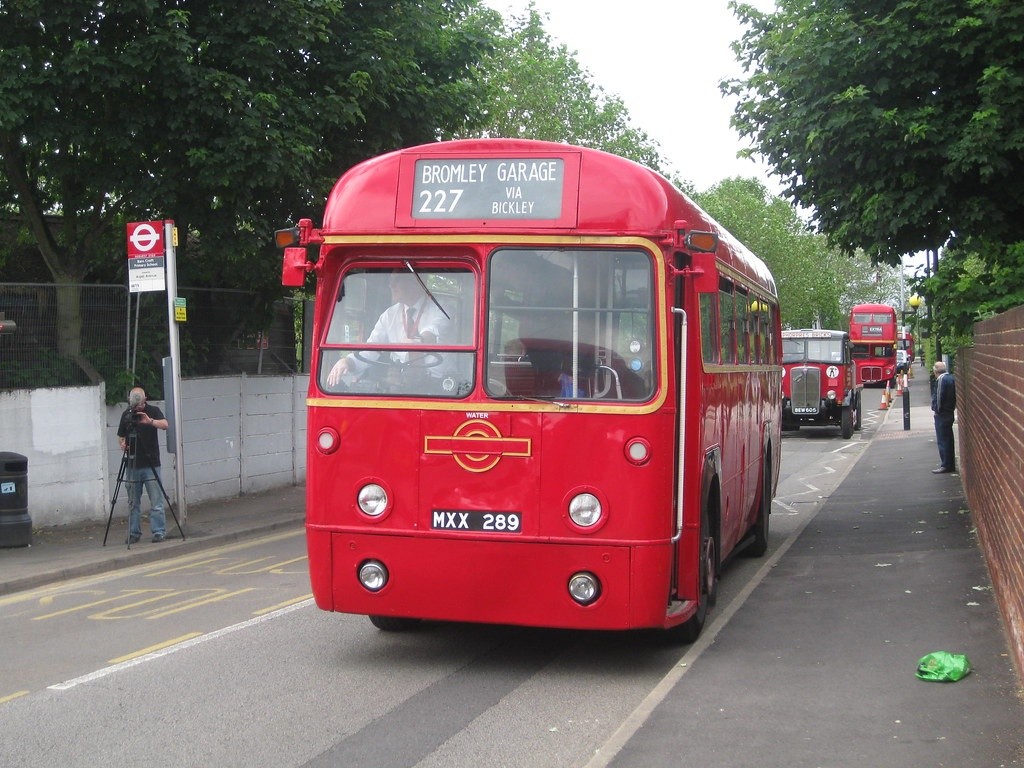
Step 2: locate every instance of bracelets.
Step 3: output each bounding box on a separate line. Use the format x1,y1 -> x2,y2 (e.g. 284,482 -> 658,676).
149,417 -> 154,426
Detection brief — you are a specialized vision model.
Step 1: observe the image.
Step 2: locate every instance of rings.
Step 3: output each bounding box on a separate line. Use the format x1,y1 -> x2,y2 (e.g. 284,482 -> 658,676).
330,374 -> 333,376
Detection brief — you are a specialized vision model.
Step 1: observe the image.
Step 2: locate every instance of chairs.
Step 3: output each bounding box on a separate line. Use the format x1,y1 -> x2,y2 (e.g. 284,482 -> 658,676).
445,303 -> 471,382
783,344 -> 800,361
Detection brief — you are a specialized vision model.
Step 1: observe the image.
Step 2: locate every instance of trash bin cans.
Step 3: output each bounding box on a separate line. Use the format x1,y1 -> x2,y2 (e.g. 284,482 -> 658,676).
0,452 -> 32,549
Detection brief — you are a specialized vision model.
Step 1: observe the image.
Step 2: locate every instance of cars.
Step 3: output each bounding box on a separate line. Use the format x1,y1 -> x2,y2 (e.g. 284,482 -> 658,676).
896,350 -> 911,373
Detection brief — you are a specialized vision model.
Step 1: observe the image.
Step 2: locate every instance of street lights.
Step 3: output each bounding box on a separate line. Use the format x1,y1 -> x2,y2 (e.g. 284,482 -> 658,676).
901,264 -> 915,311
903,294 -> 921,430
902,295 -> 921,430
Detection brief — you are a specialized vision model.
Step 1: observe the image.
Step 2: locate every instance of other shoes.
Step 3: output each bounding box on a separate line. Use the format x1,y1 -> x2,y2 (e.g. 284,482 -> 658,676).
152,535 -> 164,542
125,536 -> 139,544
932,467 -> 956,474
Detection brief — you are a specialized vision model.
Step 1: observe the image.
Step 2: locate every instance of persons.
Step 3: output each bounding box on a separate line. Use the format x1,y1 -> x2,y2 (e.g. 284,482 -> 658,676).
325,268 -> 460,389
116,386 -> 169,543
931,361 -> 957,474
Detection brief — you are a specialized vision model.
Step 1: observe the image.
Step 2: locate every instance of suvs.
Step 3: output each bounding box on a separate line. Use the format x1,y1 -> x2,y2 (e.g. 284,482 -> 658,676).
780,328 -> 862,439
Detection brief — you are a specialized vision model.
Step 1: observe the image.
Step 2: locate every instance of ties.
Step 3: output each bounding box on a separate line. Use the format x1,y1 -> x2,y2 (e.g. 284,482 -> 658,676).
406,308 -> 415,336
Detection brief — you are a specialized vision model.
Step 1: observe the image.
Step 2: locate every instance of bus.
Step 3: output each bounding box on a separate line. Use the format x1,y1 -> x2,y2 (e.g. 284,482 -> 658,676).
849,305 -> 897,387
896,332 -> 916,361
274,138 -> 784,645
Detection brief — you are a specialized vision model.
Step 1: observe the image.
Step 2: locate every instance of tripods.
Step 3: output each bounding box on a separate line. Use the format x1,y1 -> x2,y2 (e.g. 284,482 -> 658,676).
103,425 -> 186,550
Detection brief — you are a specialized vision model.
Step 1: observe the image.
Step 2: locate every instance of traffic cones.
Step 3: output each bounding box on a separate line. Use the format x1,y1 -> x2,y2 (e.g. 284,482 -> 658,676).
878,389 -> 888,410
907,372 -> 911,387
895,374 -> 903,396
884,380 -> 892,403
